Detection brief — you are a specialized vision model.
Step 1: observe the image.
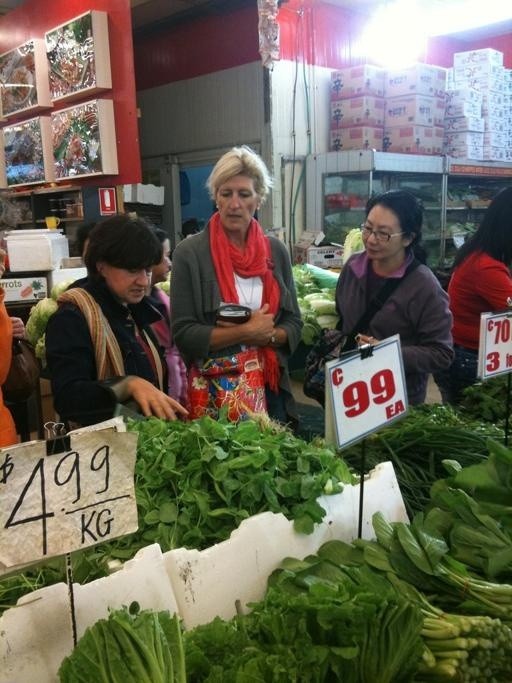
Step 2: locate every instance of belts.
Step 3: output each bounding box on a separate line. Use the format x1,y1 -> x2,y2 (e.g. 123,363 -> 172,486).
452,342 -> 478,356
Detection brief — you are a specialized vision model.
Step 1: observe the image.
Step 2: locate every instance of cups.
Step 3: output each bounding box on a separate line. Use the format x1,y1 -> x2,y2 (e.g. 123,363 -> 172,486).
45,216 -> 60,229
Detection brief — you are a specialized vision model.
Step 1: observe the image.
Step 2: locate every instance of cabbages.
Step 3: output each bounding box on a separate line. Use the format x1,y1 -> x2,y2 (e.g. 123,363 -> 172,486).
25,280 -> 74,370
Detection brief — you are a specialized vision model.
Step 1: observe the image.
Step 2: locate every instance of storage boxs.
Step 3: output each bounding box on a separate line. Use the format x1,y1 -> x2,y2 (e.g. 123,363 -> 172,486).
0,227 -> 71,302
122,181 -> 165,208
328,46 -> 512,165
293,229 -> 344,271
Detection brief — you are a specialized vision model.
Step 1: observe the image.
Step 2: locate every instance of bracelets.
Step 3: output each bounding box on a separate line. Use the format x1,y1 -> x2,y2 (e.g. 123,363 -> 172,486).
269,337 -> 275,345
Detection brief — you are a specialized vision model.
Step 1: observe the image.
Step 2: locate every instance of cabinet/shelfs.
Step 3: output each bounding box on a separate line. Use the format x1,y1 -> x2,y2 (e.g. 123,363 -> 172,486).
1,185 -> 85,256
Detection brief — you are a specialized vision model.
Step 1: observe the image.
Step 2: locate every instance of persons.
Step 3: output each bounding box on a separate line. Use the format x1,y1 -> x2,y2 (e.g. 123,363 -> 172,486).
431,186 -> 512,405
43,216 -> 189,435
335,188 -> 455,407
169,147 -> 304,434
0,251 -> 27,449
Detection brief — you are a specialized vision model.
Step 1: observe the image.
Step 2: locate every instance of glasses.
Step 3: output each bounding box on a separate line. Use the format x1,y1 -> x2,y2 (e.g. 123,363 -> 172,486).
360,222 -> 408,242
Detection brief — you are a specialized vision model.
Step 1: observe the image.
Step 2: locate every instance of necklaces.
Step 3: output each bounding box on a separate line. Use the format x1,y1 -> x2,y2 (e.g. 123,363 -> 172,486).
235,273 -> 255,306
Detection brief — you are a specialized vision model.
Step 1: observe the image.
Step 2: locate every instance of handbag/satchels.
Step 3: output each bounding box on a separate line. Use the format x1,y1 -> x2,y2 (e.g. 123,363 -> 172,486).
303,327 -> 357,410
180,351 -> 269,424
2,339 -> 41,404
